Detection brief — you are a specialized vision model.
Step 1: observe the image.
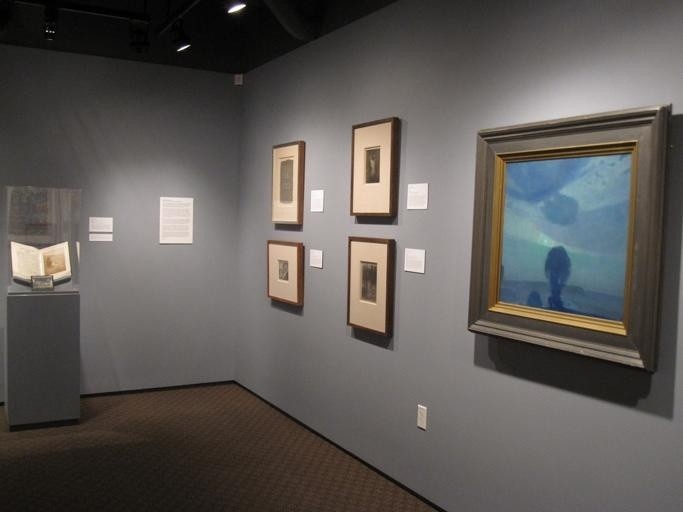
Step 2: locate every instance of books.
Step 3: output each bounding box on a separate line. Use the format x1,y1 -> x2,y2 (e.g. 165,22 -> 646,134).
8,239 -> 72,285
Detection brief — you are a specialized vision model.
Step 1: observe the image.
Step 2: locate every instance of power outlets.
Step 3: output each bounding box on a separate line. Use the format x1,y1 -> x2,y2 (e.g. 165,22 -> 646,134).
416,404 -> 427,430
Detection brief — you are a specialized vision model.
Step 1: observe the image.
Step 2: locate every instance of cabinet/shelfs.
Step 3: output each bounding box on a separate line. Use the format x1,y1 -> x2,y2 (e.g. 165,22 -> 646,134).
2,183 -> 82,430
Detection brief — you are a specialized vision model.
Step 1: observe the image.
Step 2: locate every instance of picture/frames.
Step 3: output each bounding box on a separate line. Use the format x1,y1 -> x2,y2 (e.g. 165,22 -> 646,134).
265,139 -> 307,309
346,114 -> 403,340
466,101 -> 674,373
